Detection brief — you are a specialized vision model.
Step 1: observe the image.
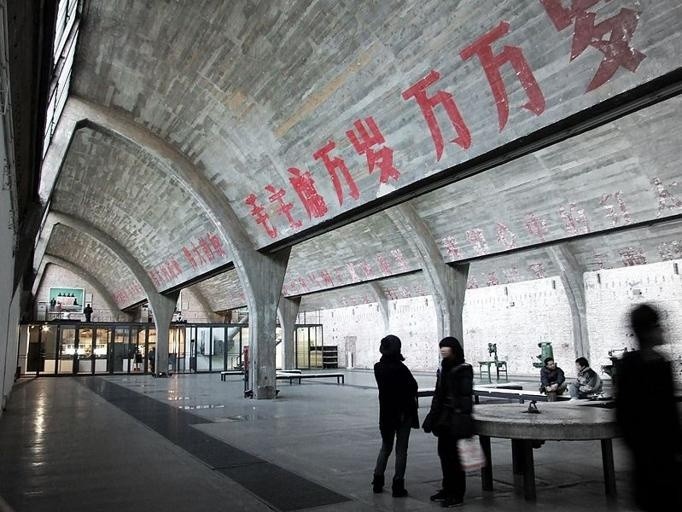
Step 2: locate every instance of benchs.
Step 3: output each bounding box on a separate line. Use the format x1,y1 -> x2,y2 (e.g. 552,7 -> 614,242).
417,382 -> 571,404
221,369 -> 344,385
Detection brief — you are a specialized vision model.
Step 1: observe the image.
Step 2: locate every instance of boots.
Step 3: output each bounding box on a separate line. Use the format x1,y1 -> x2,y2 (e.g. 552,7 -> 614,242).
392,478 -> 408,497
372,473 -> 384,493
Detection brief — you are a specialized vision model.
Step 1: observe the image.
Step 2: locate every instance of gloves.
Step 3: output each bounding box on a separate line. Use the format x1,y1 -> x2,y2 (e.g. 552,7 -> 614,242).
422,414 -> 432,433
462,415 -> 473,438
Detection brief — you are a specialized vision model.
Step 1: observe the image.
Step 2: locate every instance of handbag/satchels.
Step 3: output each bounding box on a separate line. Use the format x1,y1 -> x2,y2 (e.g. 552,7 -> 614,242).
457,434 -> 486,471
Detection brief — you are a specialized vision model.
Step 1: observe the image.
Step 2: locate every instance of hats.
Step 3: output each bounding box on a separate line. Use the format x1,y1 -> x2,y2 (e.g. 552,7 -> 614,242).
380,335 -> 400,355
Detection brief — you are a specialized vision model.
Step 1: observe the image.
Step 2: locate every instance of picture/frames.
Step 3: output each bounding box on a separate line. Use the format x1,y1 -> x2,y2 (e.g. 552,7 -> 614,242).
48,286 -> 85,315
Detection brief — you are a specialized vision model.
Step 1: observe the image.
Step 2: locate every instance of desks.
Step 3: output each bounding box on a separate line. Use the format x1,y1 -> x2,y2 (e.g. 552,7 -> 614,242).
471,401 -> 620,505
478,360 -> 508,383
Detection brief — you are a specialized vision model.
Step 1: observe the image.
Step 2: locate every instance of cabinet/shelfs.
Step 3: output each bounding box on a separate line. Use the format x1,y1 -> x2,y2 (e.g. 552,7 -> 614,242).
322,345 -> 339,368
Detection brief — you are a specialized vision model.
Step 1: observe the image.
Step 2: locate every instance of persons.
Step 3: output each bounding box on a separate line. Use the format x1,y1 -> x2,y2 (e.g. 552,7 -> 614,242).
74,299 -> 78,305
539,357 -> 567,402
610,303 -> 682,511
148,347 -> 155,375
421,337 -> 473,509
568,357 -> 602,402
371,335 -> 421,497
84,303 -> 93,322
50,298 -> 56,311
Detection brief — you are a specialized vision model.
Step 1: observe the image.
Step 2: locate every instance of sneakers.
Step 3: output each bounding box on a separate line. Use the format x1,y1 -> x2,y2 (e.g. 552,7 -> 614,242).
431,489 -> 463,507
